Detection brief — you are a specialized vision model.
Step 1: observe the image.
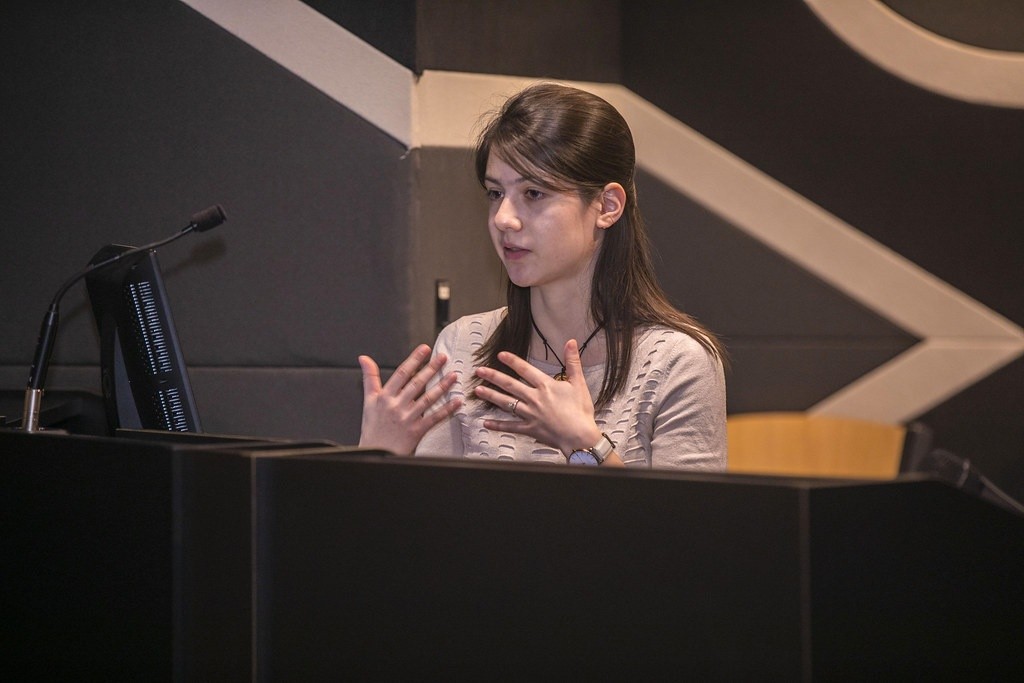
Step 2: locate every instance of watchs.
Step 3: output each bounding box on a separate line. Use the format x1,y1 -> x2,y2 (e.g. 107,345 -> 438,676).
565,432 -> 616,467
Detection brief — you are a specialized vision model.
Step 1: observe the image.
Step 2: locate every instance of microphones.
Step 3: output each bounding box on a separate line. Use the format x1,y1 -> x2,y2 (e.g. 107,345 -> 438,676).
19,203 -> 228,432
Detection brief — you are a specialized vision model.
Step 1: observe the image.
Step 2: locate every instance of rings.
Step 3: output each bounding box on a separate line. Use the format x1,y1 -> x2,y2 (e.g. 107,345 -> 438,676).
507,398 -> 521,417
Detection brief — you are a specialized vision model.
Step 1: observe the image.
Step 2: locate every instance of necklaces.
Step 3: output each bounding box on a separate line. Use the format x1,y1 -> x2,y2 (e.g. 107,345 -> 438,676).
530,310 -> 608,381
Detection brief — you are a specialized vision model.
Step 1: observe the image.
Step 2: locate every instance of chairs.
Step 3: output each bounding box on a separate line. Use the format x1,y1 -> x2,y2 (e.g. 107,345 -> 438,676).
727,412 -> 907,480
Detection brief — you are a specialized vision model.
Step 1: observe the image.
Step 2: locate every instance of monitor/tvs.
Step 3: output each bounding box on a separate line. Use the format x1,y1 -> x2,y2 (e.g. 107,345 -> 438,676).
84,241 -> 205,434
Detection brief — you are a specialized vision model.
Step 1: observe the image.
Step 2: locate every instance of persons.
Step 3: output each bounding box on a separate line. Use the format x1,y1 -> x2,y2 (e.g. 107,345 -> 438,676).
356,84 -> 727,473
